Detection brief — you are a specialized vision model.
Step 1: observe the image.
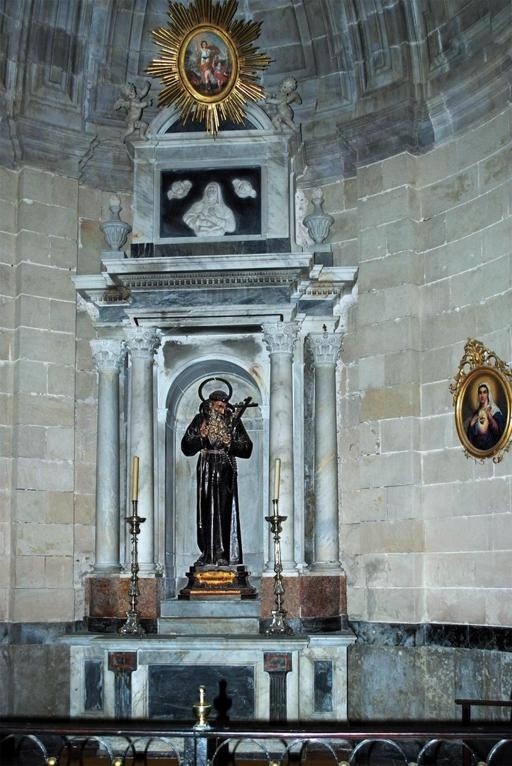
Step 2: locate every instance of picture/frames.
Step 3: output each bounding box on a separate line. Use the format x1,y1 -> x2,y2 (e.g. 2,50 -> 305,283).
455,366 -> 512,459
176,23 -> 239,106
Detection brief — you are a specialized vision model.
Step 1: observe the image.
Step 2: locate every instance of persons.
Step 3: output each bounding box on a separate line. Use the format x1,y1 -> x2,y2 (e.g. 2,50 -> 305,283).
212,60 -> 229,91
466,383 -> 504,450
266,77 -> 303,133
113,80 -> 153,144
182,182 -> 237,236
195,38 -> 214,93
181,390 -> 252,567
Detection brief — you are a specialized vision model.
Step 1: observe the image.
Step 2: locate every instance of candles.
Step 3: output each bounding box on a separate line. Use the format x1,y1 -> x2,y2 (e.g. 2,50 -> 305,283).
272,457 -> 281,500
131,455 -> 140,499
198,684 -> 205,704
